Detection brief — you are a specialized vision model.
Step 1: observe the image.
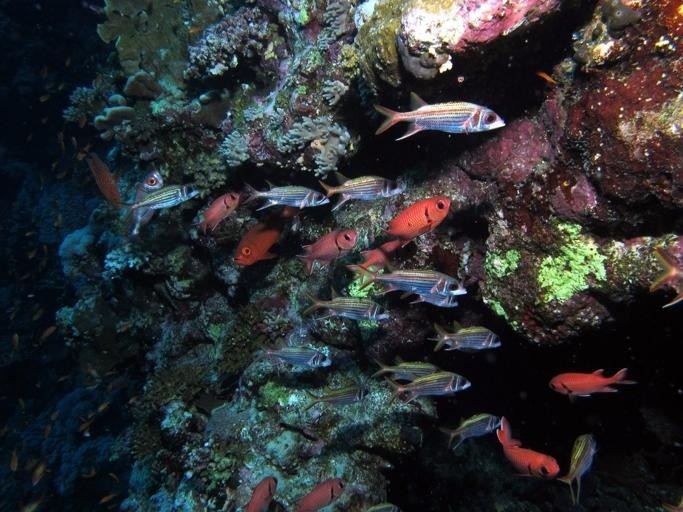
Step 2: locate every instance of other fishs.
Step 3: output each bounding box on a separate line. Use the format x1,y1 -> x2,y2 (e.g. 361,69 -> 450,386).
199,168 -> 504,412
0,1 -> 120,512
